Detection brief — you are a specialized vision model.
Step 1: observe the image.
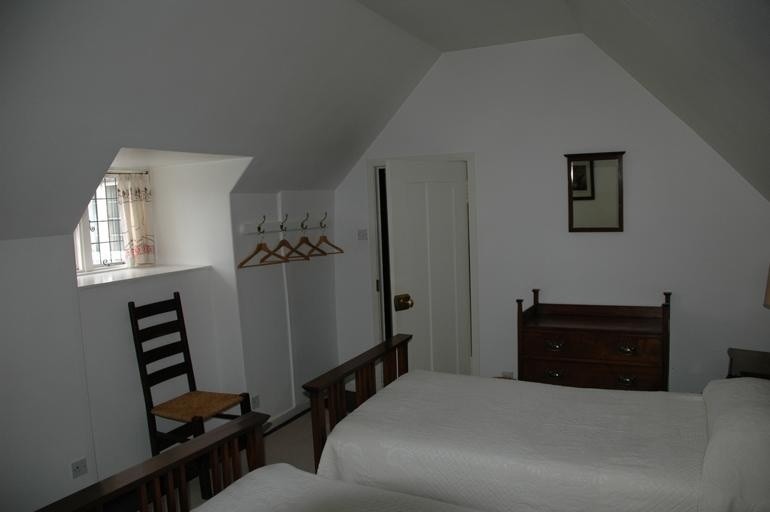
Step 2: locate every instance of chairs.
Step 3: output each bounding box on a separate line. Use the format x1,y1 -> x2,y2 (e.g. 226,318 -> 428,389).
128,290 -> 252,500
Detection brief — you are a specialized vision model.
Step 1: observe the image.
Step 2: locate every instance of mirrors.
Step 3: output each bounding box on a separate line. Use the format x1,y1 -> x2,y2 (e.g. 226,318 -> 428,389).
563,151 -> 625,232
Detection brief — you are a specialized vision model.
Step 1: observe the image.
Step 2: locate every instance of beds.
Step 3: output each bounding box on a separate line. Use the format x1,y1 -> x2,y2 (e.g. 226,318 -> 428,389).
302,333 -> 770,512
26,408 -> 478,512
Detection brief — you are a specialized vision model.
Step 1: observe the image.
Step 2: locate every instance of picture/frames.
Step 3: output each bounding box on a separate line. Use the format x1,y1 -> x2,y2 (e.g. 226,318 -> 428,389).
571,161 -> 595,200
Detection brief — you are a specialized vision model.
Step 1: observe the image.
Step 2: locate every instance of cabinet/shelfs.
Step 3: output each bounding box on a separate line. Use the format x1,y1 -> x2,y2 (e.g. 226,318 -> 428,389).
515,288 -> 672,391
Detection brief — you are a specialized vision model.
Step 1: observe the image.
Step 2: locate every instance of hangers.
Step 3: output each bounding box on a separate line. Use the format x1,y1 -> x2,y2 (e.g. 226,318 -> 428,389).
236,226 -> 344,269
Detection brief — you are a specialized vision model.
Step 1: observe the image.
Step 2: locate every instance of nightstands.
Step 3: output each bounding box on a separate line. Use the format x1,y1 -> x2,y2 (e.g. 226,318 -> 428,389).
726,347 -> 770,379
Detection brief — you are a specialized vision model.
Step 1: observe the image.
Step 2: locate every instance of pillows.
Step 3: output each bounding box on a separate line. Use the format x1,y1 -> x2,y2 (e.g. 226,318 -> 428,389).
702,378 -> 770,512
